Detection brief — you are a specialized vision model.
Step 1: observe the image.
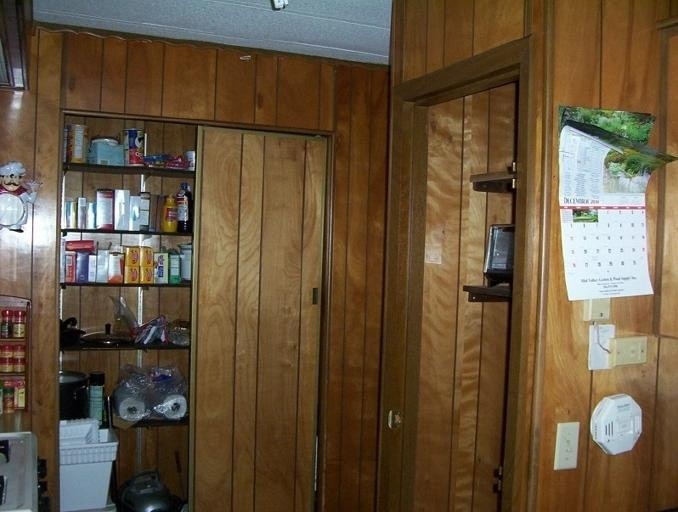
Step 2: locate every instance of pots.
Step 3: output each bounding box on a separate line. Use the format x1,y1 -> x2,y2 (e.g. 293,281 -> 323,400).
118,466 -> 170,510
59,370 -> 91,420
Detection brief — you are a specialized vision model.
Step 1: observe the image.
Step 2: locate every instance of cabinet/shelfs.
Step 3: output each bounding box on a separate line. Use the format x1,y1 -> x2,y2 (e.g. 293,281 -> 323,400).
458,169 -> 518,300
0,294 -> 33,414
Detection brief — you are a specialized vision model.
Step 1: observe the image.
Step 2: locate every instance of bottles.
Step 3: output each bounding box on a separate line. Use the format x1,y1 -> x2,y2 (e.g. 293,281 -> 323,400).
0,308 -> 26,375
177,181 -> 191,229
159,197 -> 177,232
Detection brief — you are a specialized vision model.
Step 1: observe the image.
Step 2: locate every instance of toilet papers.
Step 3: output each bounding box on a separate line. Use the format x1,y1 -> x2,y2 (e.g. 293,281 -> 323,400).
160,393 -> 187,419
114,387 -> 147,421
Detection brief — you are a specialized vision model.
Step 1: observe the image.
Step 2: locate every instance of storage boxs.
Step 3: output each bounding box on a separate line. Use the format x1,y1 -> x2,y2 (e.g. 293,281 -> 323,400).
59,428 -> 118,512
